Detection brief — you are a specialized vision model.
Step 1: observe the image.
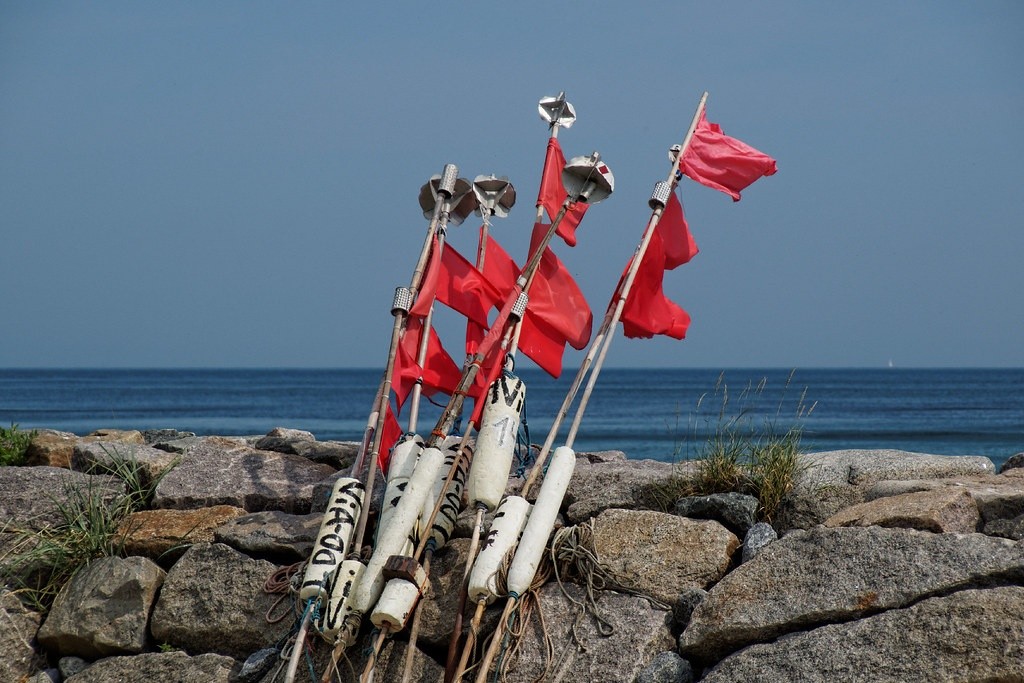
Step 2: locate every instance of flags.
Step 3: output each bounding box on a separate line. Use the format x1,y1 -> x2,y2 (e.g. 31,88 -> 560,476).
436,241 -> 502,332
481,234 -> 522,312
681,110 -> 779,203
518,222 -> 593,378
621,192 -> 702,339
392,318 -> 504,431
377,406 -> 404,482
536,138 -> 590,247
407,233 -> 441,318
